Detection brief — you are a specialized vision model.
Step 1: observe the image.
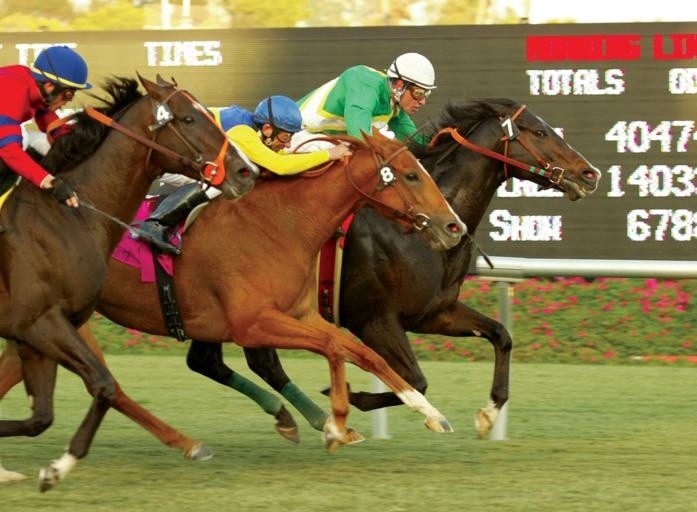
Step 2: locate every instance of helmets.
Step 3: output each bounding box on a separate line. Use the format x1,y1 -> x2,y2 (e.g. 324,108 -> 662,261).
29,46 -> 92,92
253,95 -> 304,134
386,52 -> 438,90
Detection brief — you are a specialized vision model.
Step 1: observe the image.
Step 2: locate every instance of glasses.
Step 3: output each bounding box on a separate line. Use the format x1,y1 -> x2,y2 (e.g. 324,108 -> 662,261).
409,88 -> 431,99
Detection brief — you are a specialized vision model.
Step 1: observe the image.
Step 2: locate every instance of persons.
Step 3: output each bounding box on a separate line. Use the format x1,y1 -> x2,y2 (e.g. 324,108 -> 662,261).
286,53 -> 438,146
134,95 -> 351,256
1,37 -> 91,210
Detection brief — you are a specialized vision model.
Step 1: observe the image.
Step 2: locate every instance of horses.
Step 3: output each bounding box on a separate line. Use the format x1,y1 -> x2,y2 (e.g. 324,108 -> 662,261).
0,122 -> 470,465
0,66 -> 263,496
185,94 -> 604,447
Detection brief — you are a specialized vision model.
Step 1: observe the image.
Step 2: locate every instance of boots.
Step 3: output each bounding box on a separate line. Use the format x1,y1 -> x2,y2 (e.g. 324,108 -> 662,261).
140,183 -> 213,257
